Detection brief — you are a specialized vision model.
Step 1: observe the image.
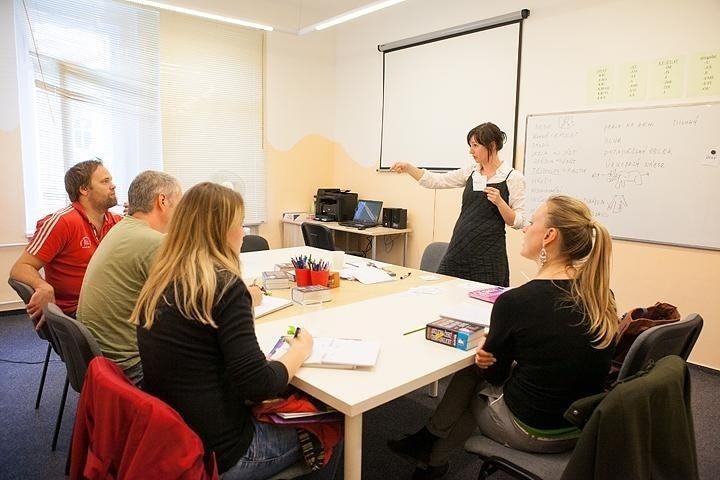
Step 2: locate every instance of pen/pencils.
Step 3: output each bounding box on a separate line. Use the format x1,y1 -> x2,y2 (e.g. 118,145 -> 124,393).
404,327 -> 425,335
294,327 -> 300,338
291,254 -> 329,271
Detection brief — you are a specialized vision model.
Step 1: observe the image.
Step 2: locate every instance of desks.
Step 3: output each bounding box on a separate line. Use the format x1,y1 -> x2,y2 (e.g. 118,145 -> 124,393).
281,214 -> 413,267
230,244 -> 514,480
243,220 -> 266,235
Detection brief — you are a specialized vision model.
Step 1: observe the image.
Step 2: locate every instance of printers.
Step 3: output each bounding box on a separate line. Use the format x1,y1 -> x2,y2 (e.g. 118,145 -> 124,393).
315,188 -> 357,221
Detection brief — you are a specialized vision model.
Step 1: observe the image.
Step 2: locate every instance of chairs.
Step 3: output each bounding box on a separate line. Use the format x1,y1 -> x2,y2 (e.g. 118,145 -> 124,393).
43,301 -> 103,454
74,348 -> 220,480
419,241 -> 451,272
462,354 -> 706,479
613,314 -> 705,386
8,276 -> 61,411
241,234 -> 269,254
300,222 -> 366,258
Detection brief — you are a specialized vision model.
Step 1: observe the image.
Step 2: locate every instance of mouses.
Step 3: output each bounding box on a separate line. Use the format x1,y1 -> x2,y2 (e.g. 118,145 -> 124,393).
358,227 -> 365,229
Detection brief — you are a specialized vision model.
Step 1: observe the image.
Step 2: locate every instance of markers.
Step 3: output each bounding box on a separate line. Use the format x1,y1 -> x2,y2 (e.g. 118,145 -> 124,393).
400,273 -> 411,278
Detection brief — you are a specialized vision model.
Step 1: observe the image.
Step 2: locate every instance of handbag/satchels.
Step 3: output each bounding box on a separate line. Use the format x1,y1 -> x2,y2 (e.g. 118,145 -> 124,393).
612,301 -> 682,362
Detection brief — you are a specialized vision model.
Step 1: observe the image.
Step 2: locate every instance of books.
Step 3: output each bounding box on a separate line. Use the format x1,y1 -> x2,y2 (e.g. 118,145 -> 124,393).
265,334 -> 382,369
261,272 -> 291,290
468,287 -> 512,304
426,317 -> 487,351
334,264 -> 394,284
253,293 -> 294,320
273,262 -> 307,273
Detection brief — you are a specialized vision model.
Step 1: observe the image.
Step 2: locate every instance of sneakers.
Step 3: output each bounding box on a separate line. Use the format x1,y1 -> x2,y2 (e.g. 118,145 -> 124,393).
387,424 -> 452,480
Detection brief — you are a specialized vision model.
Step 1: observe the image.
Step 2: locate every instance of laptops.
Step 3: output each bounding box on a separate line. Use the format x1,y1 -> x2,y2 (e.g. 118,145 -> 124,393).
338,200 -> 383,226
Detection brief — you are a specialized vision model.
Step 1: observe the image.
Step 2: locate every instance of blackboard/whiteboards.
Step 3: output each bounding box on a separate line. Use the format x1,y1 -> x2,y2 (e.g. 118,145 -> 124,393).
522,98 -> 720,250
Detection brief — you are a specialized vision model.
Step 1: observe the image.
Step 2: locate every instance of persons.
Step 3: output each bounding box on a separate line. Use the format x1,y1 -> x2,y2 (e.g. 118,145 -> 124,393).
9,161 -> 128,332
389,122 -> 528,287
386,195 -> 622,479
75,170 -> 182,390
128,182 -> 344,480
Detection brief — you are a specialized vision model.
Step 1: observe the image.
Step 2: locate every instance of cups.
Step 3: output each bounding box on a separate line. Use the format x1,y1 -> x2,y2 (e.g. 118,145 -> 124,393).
295,269 -> 330,286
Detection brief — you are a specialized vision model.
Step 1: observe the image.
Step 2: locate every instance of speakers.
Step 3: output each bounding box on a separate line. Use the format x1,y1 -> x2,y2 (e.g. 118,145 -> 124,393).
383,207 -> 392,227
391,208 -> 407,229
